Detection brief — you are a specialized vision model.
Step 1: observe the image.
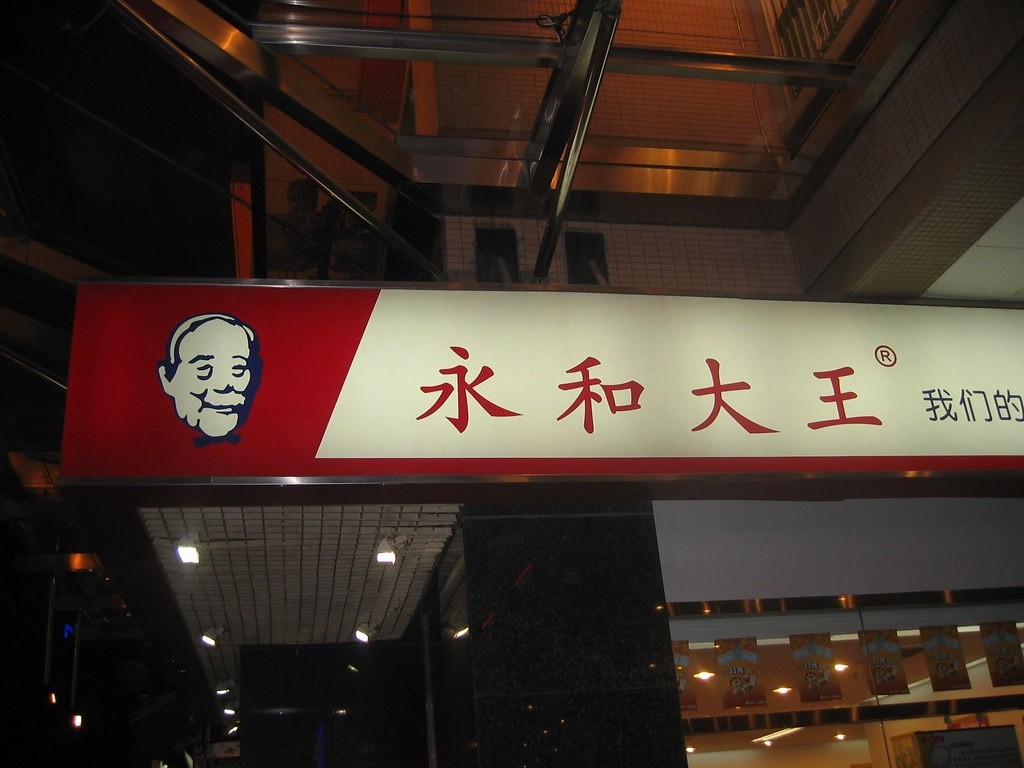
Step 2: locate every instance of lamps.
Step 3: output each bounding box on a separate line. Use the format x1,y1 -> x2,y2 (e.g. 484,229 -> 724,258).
215,679 -> 236,695
355,622 -> 380,643
375,536 -> 397,564
201,625 -> 226,647
178,532 -> 202,564
223,700 -> 240,715
